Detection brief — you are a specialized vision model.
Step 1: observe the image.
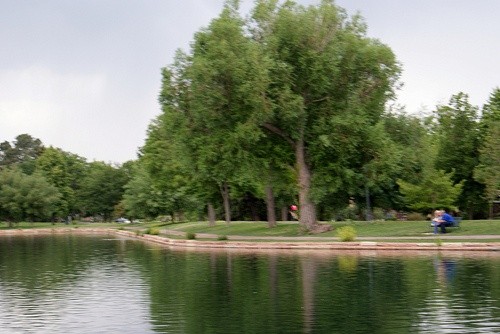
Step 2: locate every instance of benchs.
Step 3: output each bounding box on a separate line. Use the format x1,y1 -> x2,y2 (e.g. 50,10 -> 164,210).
429,216 -> 461,233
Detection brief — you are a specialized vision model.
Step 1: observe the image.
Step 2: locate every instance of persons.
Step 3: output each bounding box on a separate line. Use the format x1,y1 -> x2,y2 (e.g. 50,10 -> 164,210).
204,204 -> 209,216
432,210 -> 445,232
385,208 -> 393,218
395,210 -> 402,218
122,216 -> 133,222
438,209 -> 455,233
336,214 -> 346,222
